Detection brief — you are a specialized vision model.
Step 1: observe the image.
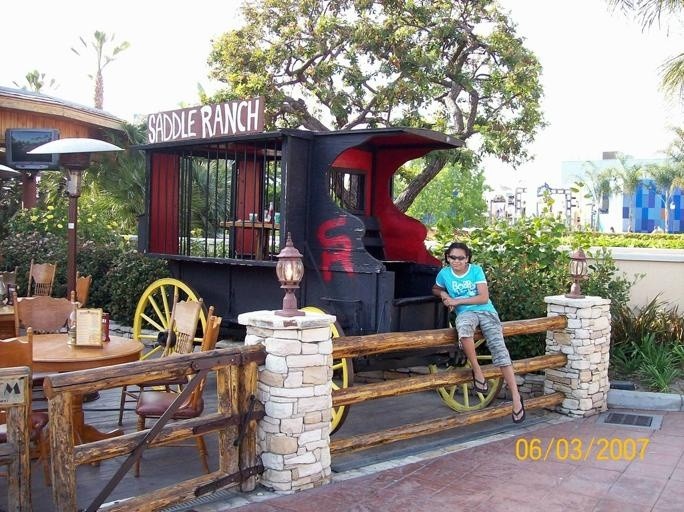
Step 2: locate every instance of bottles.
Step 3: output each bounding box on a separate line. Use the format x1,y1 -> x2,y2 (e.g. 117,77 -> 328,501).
67,290 -> 79,344
0,275 -> 9,307
264,209 -> 269,223
267,202 -> 274,224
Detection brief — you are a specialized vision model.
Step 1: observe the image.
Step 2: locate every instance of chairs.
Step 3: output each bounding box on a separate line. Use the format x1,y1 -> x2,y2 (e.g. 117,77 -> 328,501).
118,299 -> 222,478
2,328 -> 54,484
0,259 -> 91,336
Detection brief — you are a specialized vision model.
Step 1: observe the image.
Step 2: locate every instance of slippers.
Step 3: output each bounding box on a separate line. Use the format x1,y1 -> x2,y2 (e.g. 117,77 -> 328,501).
472,366 -> 487,393
510,394 -> 525,423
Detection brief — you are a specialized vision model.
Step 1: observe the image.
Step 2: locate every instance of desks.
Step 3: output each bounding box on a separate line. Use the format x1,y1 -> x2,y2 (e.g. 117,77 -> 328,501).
4,333 -> 145,467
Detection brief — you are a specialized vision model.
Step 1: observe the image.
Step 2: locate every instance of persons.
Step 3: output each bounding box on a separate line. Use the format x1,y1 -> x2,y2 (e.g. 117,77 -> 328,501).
431,242 -> 526,424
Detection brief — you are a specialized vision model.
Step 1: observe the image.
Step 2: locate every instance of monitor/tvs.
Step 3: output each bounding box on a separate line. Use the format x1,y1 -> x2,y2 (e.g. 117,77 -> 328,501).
6,128 -> 61,172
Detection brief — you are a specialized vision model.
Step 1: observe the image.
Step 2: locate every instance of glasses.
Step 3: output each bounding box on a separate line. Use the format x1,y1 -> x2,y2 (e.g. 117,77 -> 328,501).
448,255 -> 466,260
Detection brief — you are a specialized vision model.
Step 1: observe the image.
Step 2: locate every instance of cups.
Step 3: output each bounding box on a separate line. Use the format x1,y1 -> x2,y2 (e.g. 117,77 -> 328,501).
249,213 -> 259,224
275,212 -> 280,225
